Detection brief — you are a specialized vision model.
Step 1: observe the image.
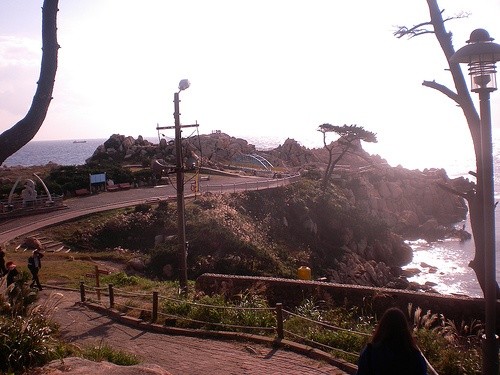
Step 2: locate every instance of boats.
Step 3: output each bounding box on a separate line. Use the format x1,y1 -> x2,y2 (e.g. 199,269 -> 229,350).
73,140 -> 87,144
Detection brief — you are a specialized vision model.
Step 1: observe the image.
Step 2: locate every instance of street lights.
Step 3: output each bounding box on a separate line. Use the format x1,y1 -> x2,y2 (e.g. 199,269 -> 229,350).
449,28 -> 500,374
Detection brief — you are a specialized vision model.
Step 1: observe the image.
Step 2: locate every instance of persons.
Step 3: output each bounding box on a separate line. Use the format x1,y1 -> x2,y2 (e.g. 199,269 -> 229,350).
356,308 -> 427,375
27,250 -> 44,292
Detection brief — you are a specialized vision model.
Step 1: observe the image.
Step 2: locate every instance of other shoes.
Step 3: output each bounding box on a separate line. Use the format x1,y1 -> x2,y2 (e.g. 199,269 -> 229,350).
30,285 -> 33,288
38,285 -> 41,288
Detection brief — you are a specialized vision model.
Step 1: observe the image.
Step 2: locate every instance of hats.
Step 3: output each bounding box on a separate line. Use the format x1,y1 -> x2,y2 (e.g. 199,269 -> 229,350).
6,262 -> 16,267
